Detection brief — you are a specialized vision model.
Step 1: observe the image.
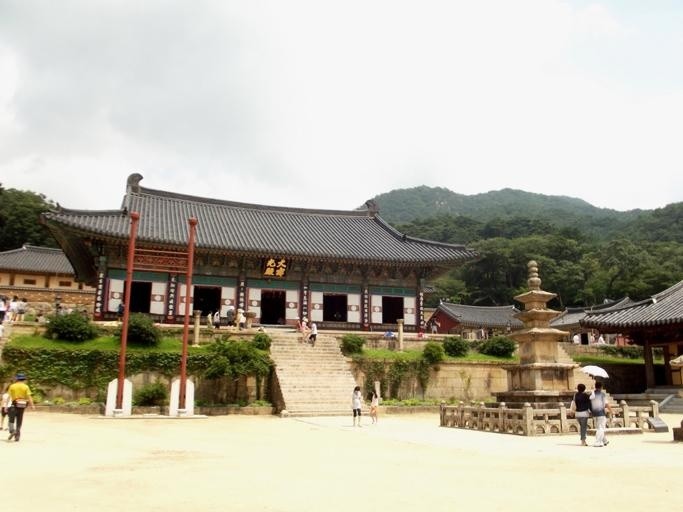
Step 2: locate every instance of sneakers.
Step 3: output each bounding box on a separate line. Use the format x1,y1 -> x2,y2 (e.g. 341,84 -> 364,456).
578,439 -> 610,448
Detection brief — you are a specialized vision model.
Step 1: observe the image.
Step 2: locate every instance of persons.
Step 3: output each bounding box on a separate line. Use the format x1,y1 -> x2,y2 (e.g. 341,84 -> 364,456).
0,294 -> 88,343
294,315 -> 317,347
6,372 -> 35,442
571,332 -> 581,345
416,315 -> 441,339
383,328 -> 397,341
506,318 -> 512,334
0,382 -> 11,431
369,389 -> 378,424
114,300 -> 125,324
597,333 -> 606,345
570,383 -> 592,446
587,381 -> 613,447
589,332 -> 595,344
206,304 -> 245,332
351,386 -> 363,425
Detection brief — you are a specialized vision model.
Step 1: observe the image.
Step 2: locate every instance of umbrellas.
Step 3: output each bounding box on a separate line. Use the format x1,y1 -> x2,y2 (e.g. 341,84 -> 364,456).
579,364 -> 610,392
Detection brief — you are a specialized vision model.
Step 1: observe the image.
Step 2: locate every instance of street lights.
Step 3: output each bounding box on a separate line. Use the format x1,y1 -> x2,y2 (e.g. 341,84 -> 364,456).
55,294 -> 62,320
507,324 -> 511,334
